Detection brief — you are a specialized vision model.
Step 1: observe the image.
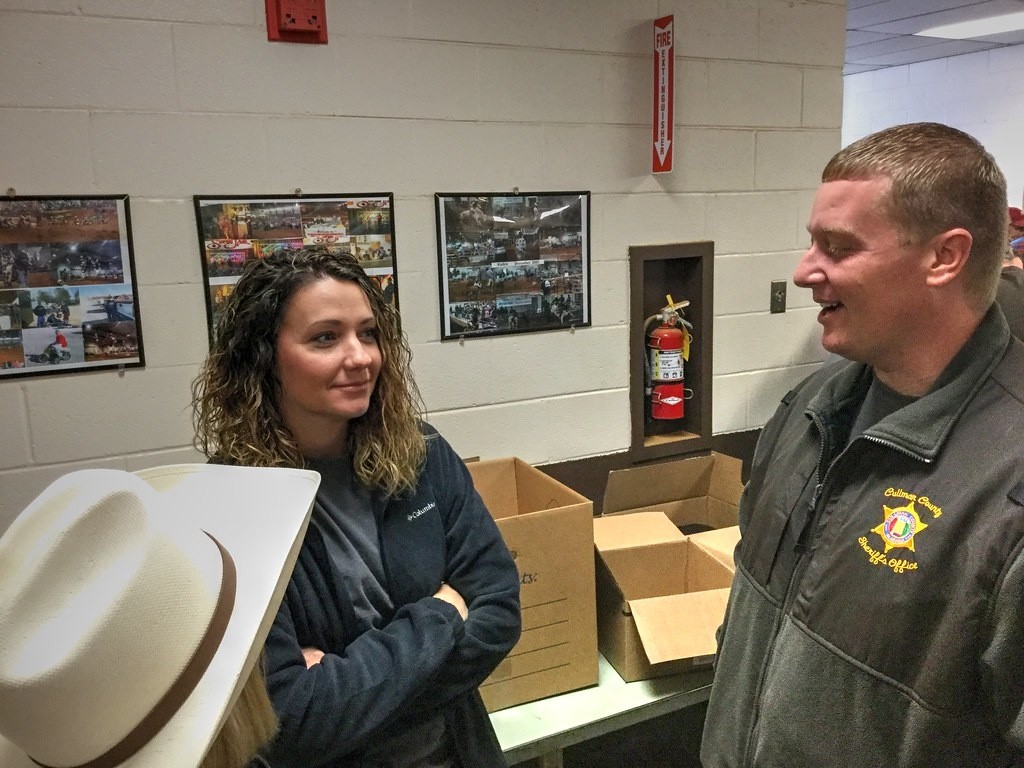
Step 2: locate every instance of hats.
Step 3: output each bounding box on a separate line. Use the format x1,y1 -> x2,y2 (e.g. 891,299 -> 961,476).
1008,208 -> 1024,228
0,464 -> 322,768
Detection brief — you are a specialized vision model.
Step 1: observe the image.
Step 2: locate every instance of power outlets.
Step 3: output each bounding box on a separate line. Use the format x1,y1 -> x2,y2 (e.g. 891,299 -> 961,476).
769,281 -> 789,312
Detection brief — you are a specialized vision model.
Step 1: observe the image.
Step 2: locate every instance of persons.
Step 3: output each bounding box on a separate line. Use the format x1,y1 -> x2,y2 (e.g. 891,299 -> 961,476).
447,231 -> 582,263
105,295 -> 120,322
250,215 -> 300,230
10,246 -> 29,288
187,245 -> 522,768
450,263 -> 583,300
32,299 -> 70,327
541,295 -> 572,319
698,123 -> 1024,768
363,211 -> 382,231
452,294 -> 519,331
204,213 -> 238,238
90,331 -> 135,356
50,329 -> 68,359
357,246 -> 392,261
1,212 -> 109,229
448,197 -> 494,241
313,214 -> 341,225
0,463 -> 321,767
57,256 -> 98,282
209,254 -> 240,269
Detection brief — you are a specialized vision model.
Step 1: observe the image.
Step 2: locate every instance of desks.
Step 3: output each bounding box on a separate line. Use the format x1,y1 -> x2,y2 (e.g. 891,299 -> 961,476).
487,653 -> 714,768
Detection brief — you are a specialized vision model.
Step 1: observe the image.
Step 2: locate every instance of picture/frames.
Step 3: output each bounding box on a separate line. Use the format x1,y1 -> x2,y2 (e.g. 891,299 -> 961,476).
434,192 -> 593,342
194,191 -> 405,356
0,195 -> 146,377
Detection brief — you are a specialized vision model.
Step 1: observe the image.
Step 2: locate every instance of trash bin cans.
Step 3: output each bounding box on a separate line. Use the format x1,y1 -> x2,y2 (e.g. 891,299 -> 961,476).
644,295 -> 693,419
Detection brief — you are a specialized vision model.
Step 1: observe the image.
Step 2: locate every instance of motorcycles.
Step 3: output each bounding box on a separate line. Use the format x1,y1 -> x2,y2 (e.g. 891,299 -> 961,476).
38,346 -> 70,363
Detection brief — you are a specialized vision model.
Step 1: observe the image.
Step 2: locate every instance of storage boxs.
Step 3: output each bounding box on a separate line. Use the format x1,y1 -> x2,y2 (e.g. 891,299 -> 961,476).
603,447 -> 743,530
465,458 -> 599,711
592,509 -> 742,683
